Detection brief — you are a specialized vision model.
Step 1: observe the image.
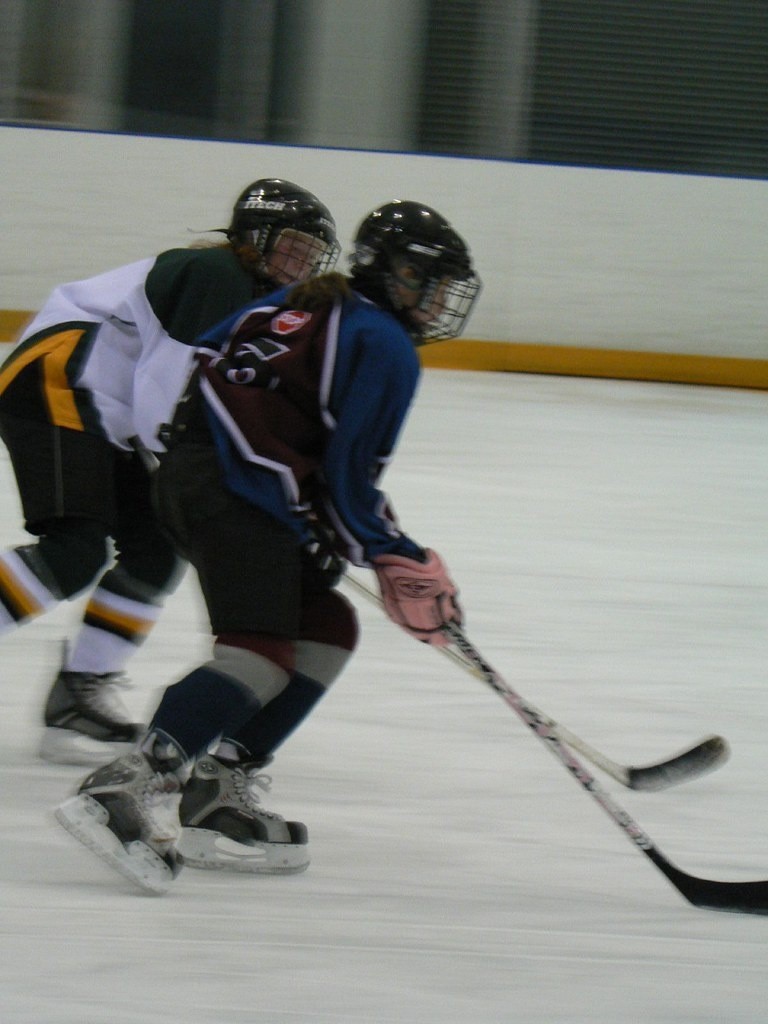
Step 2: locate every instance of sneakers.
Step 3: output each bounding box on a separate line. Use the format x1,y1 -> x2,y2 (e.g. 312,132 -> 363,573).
174,752 -> 310,874
57,727 -> 192,894
39,638 -> 147,766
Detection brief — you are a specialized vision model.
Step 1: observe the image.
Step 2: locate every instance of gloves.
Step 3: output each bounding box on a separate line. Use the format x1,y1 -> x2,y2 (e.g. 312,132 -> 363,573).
369,548 -> 464,646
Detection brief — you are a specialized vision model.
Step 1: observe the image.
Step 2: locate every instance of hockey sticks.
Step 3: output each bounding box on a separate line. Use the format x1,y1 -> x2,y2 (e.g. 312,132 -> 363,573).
440,609 -> 768,919
341,575 -> 733,794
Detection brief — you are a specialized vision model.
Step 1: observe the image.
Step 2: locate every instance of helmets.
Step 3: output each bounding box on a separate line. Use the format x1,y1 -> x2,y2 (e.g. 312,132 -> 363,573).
350,201 -> 472,301
227,178 -> 336,256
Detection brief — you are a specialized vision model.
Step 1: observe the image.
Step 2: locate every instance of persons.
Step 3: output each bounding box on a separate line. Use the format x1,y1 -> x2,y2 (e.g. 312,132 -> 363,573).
0,177 -> 345,752
54,196 -> 481,898
